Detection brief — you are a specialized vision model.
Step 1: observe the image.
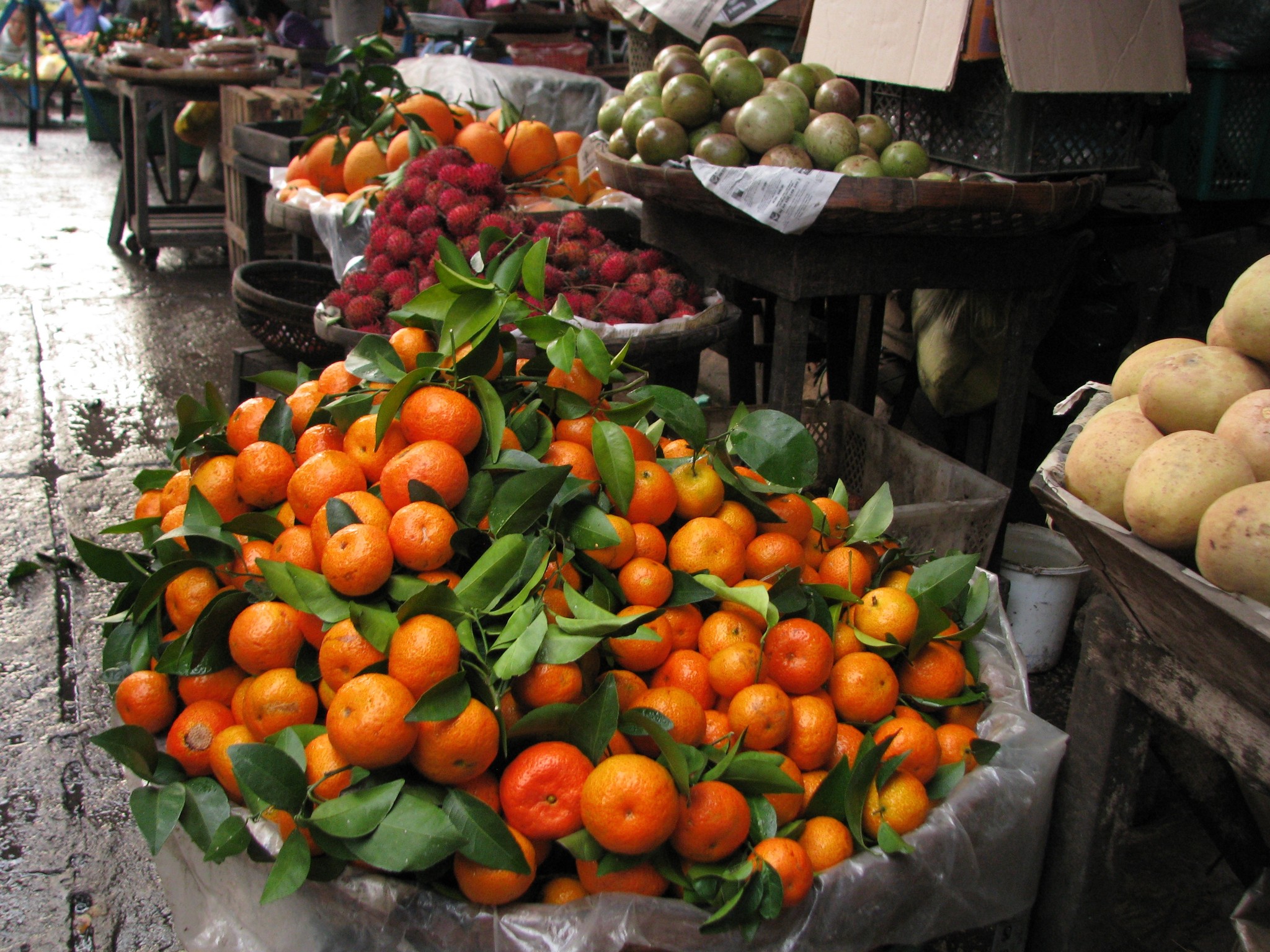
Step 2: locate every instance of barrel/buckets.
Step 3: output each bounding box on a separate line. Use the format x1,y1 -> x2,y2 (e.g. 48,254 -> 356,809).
995,521 -> 1092,672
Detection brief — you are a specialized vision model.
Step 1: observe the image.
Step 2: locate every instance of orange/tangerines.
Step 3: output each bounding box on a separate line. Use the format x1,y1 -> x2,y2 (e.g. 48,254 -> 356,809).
93,13 -> 218,54
113,329 -> 989,913
275,89 -> 619,203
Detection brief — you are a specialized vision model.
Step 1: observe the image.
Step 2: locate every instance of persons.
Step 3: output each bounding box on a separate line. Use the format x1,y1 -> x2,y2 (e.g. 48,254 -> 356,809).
47,0 -> 112,40
0,5 -> 42,67
175,0 -> 252,38
248,0 -> 339,76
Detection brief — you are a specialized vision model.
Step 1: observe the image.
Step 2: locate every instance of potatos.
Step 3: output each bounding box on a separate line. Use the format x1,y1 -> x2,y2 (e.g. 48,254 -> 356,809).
1061,255 -> 1269,614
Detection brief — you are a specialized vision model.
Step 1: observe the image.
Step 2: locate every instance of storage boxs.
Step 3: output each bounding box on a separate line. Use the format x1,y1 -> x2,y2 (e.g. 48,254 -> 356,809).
722,398 -> 1011,568
802,0 -> 1195,176
